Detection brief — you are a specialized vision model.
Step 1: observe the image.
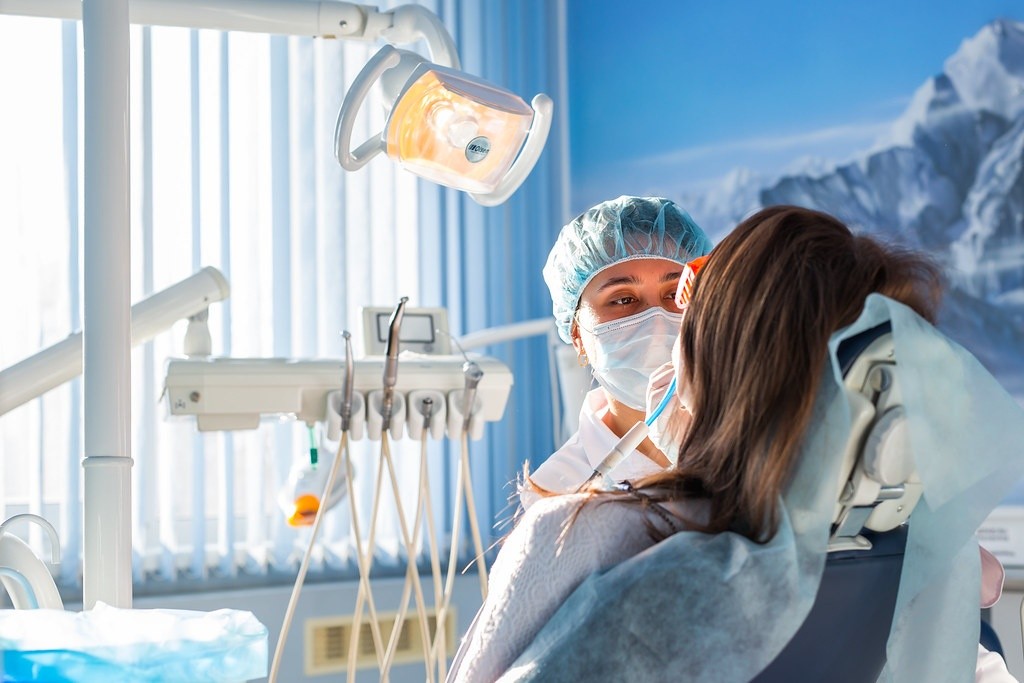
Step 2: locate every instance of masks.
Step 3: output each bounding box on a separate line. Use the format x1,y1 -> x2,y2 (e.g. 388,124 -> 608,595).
574,306 -> 684,411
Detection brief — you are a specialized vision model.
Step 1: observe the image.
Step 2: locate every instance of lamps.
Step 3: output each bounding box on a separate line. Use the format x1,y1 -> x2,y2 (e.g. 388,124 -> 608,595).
380,57 -> 534,193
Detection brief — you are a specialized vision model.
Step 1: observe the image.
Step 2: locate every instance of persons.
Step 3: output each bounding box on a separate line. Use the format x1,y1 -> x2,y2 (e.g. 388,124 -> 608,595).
445,206 -> 934,683
520,195 -> 1020,683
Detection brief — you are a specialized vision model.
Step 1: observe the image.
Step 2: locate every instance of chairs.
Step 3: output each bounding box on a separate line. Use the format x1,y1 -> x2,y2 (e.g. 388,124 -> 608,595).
487,312 -> 1023,683
